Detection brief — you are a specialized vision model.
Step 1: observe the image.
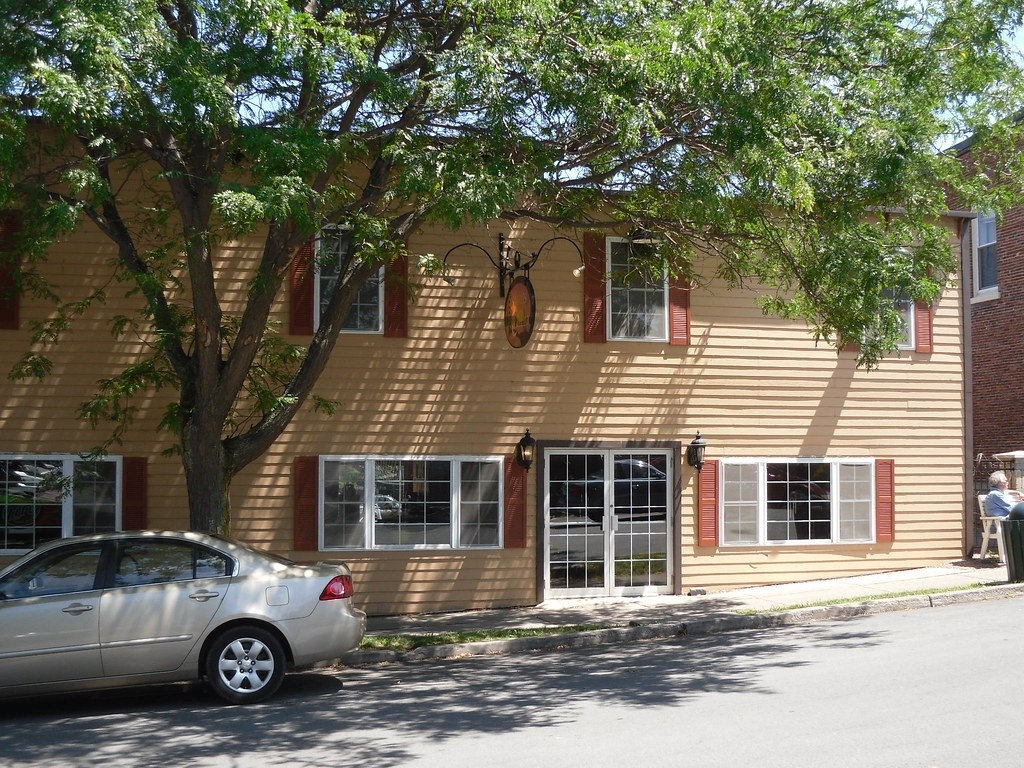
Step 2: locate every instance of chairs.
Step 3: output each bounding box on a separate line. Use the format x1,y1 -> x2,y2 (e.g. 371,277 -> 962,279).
979,495 -> 1005,562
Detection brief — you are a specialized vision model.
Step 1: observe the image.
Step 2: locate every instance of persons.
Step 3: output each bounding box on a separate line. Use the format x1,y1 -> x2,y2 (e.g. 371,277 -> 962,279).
985,471 -> 1024,517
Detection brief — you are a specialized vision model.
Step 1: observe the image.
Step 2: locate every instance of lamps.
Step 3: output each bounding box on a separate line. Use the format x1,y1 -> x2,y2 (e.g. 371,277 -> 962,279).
442,232 -> 586,297
517,428 -> 536,473
687,431 -> 706,474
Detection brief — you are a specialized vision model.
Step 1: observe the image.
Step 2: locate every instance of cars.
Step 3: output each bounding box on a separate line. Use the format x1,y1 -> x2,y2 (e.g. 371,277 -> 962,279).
562,459 -> 670,520
1,528 -> 368,702
765,464 -> 823,508
375,495 -> 402,509
